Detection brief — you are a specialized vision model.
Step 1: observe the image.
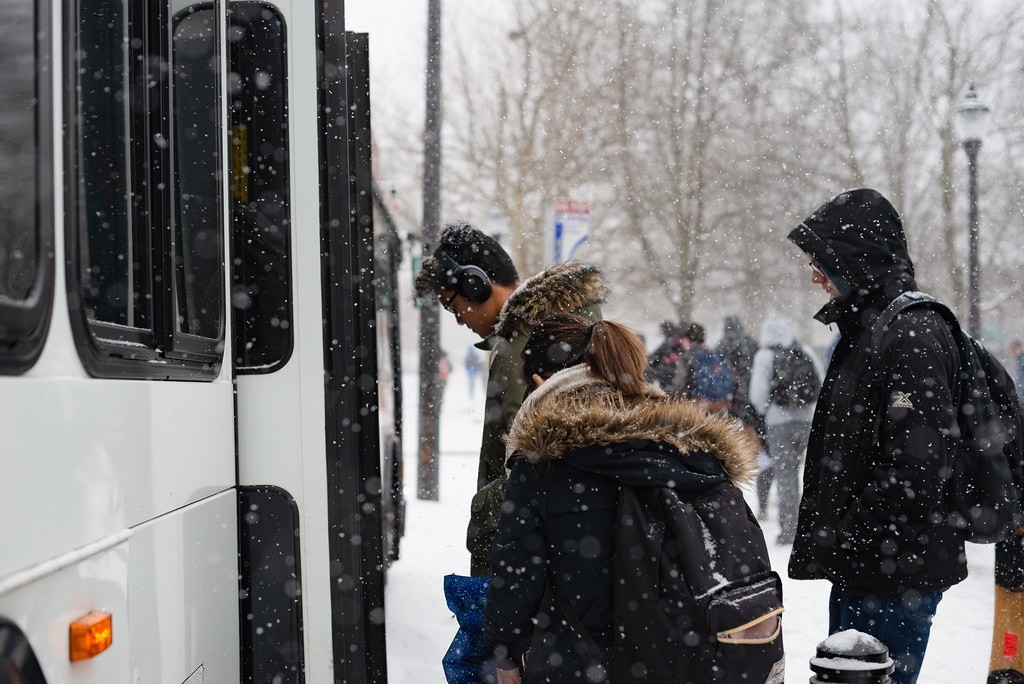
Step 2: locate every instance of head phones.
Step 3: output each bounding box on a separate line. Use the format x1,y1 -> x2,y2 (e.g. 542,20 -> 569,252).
434,247 -> 493,304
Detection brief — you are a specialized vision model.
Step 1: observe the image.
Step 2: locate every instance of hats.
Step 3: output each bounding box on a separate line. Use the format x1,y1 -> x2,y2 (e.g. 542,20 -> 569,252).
679,323 -> 705,344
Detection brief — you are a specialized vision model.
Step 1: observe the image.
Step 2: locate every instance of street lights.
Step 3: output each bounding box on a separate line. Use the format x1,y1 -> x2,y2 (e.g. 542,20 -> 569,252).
950,80 -> 991,341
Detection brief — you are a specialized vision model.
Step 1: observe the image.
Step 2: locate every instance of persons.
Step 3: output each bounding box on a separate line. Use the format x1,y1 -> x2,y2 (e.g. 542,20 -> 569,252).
669,321 -> 730,417
464,345 -> 482,396
1010,339 -> 1024,405
438,345 -> 451,411
643,319 -> 685,389
485,311 -> 785,684
750,310 -> 825,544
716,313 -> 761,424
786,187 -> 967,684
410,223 -> 610,575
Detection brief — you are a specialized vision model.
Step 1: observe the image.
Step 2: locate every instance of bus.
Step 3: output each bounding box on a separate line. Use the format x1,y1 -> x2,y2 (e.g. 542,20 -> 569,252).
0,0 -> 441,683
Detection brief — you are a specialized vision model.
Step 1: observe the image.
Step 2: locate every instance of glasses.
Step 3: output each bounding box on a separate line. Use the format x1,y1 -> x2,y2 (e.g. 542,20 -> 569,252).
807,262 -> 824,279
441,291 -> 460,314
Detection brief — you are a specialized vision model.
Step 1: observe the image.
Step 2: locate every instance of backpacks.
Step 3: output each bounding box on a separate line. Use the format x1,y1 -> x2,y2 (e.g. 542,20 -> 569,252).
514,449 -> 785,684
684,349 -> 734,406
868,288 -> 1023,545
769,341 -> 822,410
